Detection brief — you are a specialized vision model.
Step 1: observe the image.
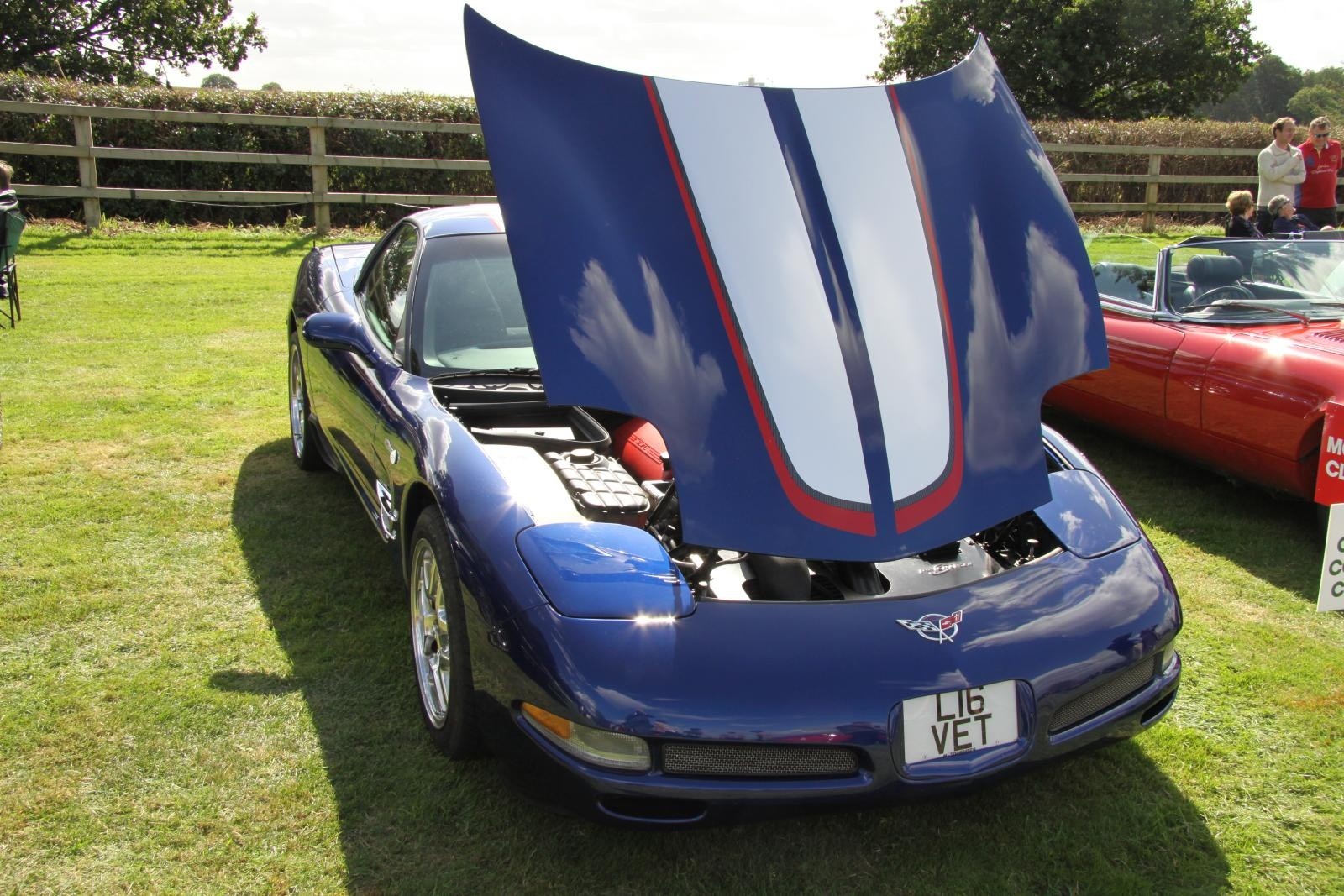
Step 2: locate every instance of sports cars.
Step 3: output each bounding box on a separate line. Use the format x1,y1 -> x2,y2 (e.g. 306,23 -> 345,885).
1041,222 -> 1344,526
283,2 -> 1187,832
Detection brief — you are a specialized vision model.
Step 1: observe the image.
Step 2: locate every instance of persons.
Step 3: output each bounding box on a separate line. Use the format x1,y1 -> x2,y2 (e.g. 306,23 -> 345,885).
1295,117 -> 1344,230
0,159 -> 20,219
433,259 -> 481,347
1255,116 -> 1306,234
1225,190 -> 1266,277
1267,194 -> 1336,241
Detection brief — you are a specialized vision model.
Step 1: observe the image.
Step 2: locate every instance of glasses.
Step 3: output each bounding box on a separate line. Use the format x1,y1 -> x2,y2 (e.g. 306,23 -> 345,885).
1312,133 -> 1329,139
1278,198 -> 1290,209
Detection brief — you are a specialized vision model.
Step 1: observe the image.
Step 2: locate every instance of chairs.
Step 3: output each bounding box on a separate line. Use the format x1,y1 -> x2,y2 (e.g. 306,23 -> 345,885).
1180,253 -> 1258,306
1093,262 -> 1153,309
0,209 -> 27,330
1219,211 -> 1344,240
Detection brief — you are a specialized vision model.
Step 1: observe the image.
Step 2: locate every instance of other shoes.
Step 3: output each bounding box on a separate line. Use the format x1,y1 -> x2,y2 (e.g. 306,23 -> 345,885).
0,277 -> 9,299
